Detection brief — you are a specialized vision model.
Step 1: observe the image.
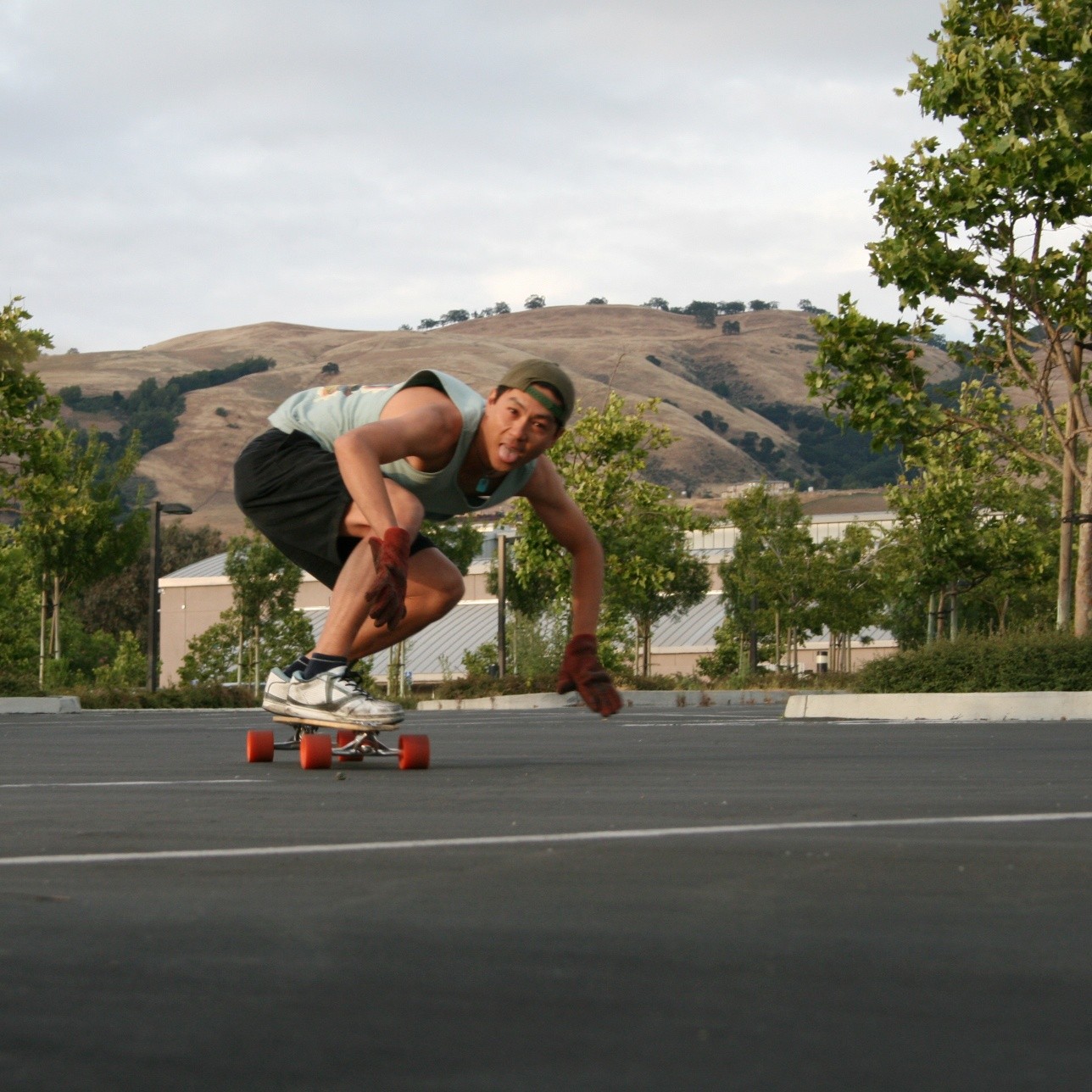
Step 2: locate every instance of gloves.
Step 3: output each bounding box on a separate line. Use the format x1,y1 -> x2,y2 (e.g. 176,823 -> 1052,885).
364,526 -> 411,632
556,634 -> 624,718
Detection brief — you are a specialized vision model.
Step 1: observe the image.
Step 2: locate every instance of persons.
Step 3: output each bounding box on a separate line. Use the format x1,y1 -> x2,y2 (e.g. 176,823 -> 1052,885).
234,358 -> 624,725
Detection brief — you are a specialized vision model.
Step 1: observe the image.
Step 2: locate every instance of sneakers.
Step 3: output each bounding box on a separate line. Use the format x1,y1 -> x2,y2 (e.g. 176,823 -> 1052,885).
262,667 -> 291,716
285,658 -> 406,724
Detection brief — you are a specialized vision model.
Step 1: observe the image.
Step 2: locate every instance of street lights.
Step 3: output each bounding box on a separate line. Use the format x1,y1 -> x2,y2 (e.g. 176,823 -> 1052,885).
146,500 -> 193,696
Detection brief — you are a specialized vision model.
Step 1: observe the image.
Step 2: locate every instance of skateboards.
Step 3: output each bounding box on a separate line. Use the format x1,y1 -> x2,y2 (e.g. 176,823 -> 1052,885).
246,714 -> 431,773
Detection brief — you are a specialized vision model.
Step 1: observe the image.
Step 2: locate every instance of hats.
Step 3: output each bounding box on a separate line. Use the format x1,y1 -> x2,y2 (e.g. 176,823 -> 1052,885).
497,359 -> 575,428
